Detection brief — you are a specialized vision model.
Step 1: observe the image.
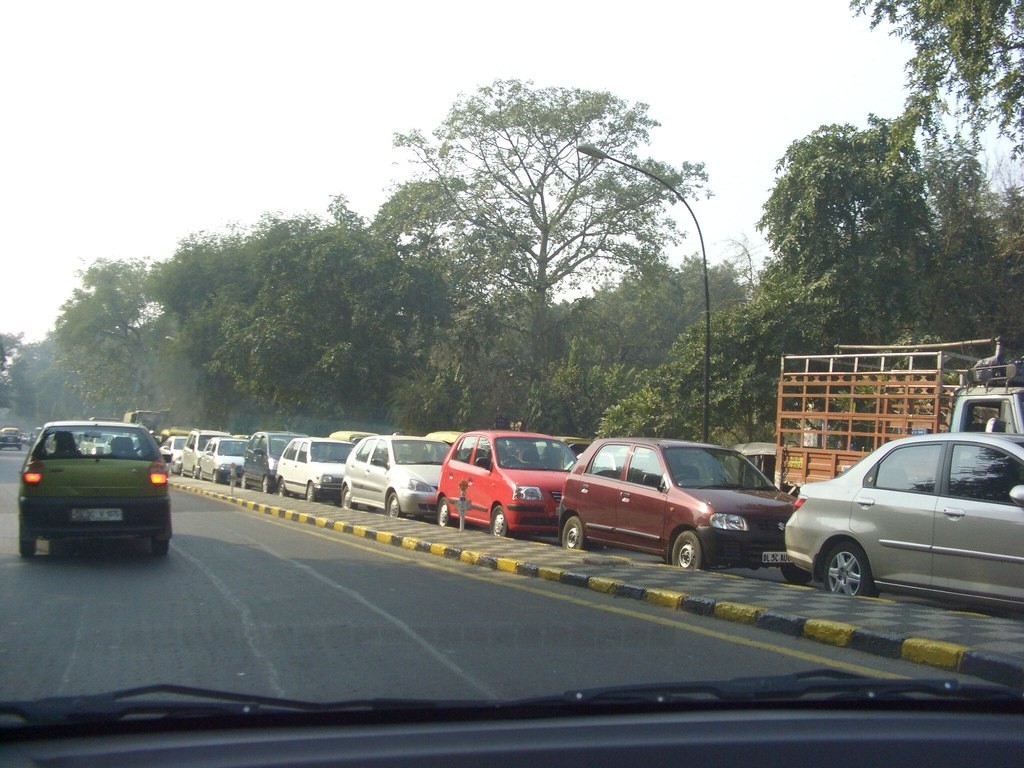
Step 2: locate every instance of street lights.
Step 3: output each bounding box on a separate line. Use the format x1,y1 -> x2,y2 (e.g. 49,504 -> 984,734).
576,140 -> 709,439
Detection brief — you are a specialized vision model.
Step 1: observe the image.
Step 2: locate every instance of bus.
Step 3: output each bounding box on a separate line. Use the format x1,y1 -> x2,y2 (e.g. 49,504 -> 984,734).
124,409 -> 170,444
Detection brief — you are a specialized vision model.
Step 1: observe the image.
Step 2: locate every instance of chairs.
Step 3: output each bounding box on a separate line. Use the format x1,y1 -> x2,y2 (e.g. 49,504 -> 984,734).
105,436 -> 140,458
46,432 -> 82,458
465,444 -> 561,468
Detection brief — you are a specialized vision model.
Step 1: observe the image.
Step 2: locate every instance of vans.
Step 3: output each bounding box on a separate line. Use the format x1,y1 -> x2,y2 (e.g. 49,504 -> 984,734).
422,429 -> 469,455
531,434 -> 615,477
326,430 -> 380,458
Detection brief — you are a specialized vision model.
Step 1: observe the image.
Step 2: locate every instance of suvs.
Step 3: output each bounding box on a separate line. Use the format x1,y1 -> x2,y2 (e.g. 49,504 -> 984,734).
181,428 -> 232,479
241,431 -> 303,493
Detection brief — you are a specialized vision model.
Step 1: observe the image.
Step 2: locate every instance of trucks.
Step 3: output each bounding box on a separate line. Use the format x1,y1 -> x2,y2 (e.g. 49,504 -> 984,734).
764,337 -> 1024,488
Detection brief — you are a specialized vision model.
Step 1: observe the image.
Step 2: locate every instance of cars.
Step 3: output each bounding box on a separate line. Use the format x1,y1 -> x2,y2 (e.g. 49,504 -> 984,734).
782,431 -> 1024,614
435,429 -> 581,540
159,428 -> 190,444
276,436 -> 358,502
341,434 -> 457,519
199,438 -> 250,484
158,435 -> 188,474
16,420 -> 173,559
19,427 -> 54,447
555,437 -> 815,585
0,428 -> 23,450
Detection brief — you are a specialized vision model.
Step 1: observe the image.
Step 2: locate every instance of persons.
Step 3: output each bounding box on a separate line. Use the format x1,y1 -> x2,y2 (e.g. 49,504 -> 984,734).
53,431 -> 74,451
488,440 -> 526,466
